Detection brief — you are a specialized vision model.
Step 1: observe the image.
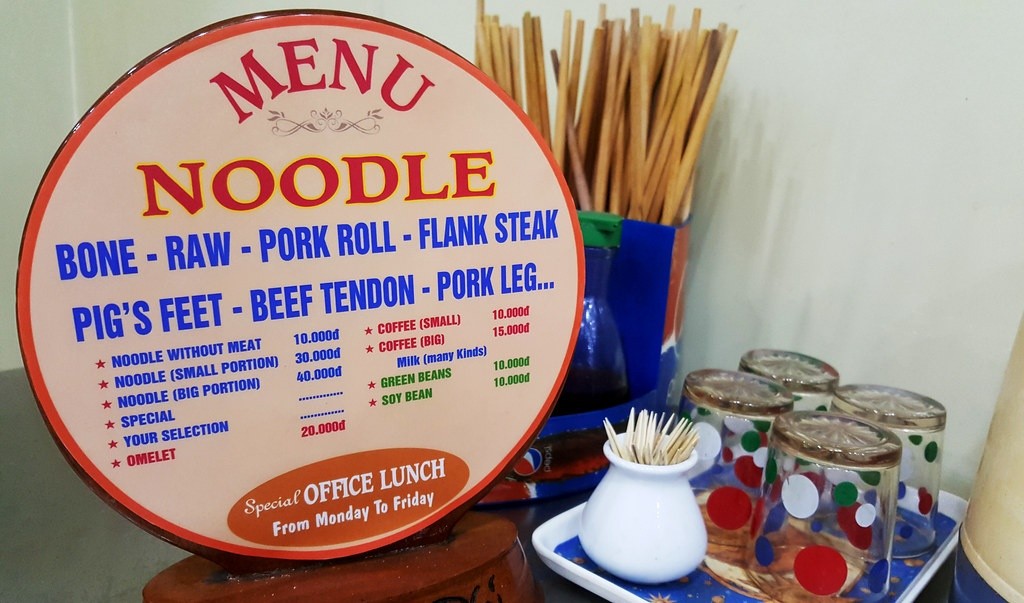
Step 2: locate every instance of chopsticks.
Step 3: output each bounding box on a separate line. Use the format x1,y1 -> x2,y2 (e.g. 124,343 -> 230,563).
468,0 -> 735,230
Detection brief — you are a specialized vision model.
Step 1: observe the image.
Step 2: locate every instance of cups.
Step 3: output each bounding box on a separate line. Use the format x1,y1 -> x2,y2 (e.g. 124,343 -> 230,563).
675,347 -> 947,601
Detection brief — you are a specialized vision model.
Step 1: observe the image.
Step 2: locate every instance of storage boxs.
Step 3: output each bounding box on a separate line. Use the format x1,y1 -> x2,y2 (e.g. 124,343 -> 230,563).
560,211 -> 695,408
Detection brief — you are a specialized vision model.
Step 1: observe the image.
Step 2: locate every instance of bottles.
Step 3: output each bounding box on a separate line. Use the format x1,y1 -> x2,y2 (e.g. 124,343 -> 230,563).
555,209 -> 628,470
583,433 -> 706,582
